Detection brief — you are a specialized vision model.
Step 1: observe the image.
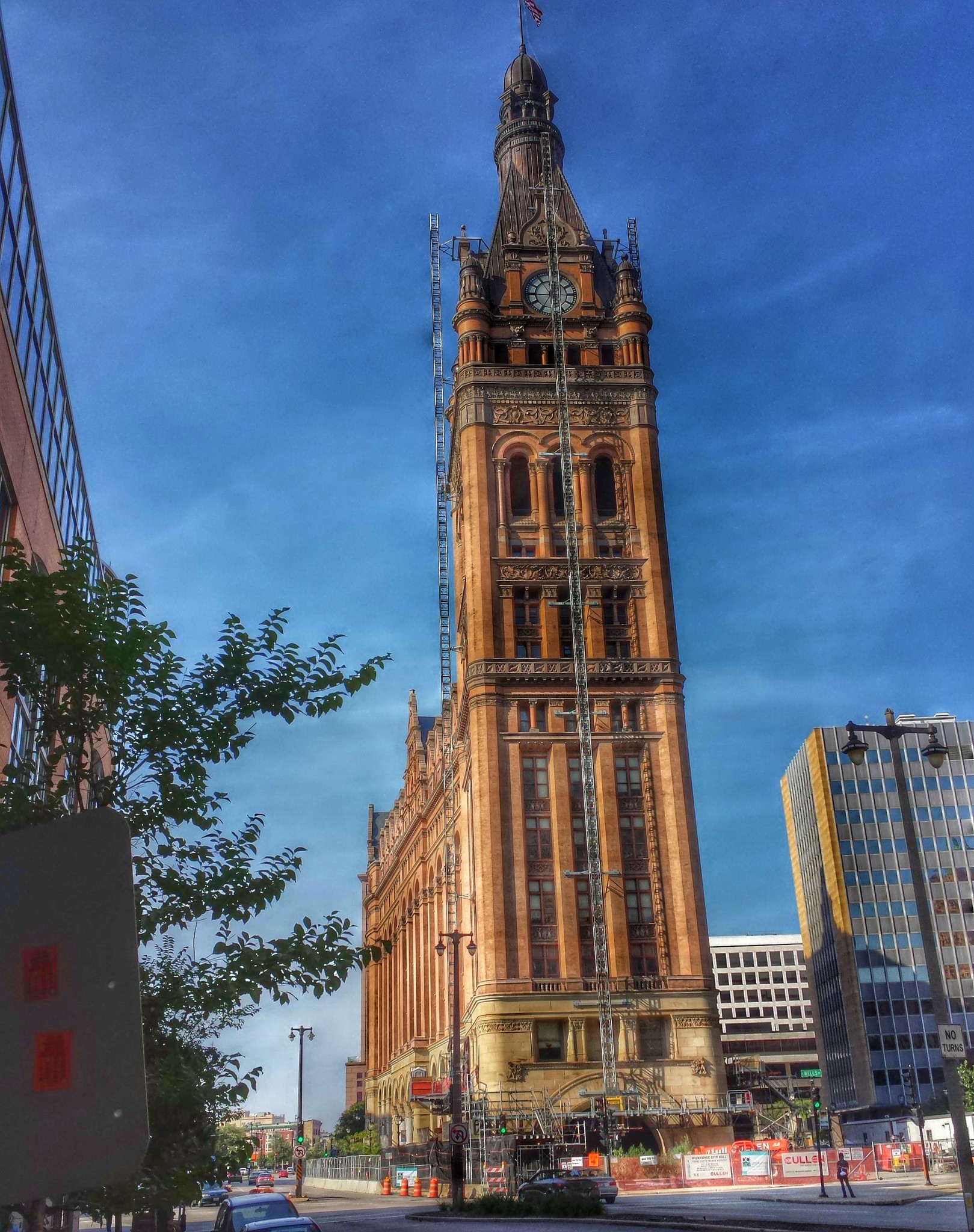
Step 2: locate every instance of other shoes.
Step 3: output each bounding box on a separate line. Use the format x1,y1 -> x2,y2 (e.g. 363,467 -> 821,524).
852,1196 -> 856,1198
843,1196 -> 847,1198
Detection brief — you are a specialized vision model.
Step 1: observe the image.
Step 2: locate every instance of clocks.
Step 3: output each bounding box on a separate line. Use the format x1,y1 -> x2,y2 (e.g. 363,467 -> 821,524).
522,268 -> 581,316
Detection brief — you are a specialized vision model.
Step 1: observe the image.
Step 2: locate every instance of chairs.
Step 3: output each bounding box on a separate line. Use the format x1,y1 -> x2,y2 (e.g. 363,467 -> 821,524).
564,1173 -> 572,1177
545,1173 -> 553,1178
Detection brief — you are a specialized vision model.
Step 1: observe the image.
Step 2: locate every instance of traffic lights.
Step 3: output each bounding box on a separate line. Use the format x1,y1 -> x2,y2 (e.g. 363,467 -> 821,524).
902,1072 -> 916,1108
297,1124 -> 304,1144
324,1152 -> 328,1157
595,1096 -> 605,1118
812,1087 -> 822,1110
607,1109 -> 617,1132
499,1114 -> 506,1134
911,1109 -> 921,1127
248,1158 -> 251,1161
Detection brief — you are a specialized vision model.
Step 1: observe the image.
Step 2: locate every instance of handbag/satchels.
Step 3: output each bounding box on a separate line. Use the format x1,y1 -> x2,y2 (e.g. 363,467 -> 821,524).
840,1168 -> 846,1176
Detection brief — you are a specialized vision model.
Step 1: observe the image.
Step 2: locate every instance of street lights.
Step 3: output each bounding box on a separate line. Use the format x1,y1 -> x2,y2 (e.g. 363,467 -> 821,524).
367,1121 -> 374,1154
289,1026 -> 315,1198
433,929 -> 479,1214
272,1136 -> 279,1174
839,708 -> 974,1232
244,1123 -> 258,1175
394,1113 -> 403,1147
328,1133 -> 334,1159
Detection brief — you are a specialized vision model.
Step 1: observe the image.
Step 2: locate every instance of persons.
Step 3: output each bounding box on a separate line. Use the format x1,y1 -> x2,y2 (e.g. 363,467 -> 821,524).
837,1153 -> 856,1198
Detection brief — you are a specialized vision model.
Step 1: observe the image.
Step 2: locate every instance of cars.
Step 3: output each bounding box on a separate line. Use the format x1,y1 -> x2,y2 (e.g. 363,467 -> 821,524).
227,1168 -> 243,1183
517,1168 -> 619,1205
191,1181 -> 230,1207
240,1216 -> 322,1232
286,1167 -> 295,1175
278,1170 -> 288,1179
211,1193 -> 300,1232
239,1168 -> 281,1188
